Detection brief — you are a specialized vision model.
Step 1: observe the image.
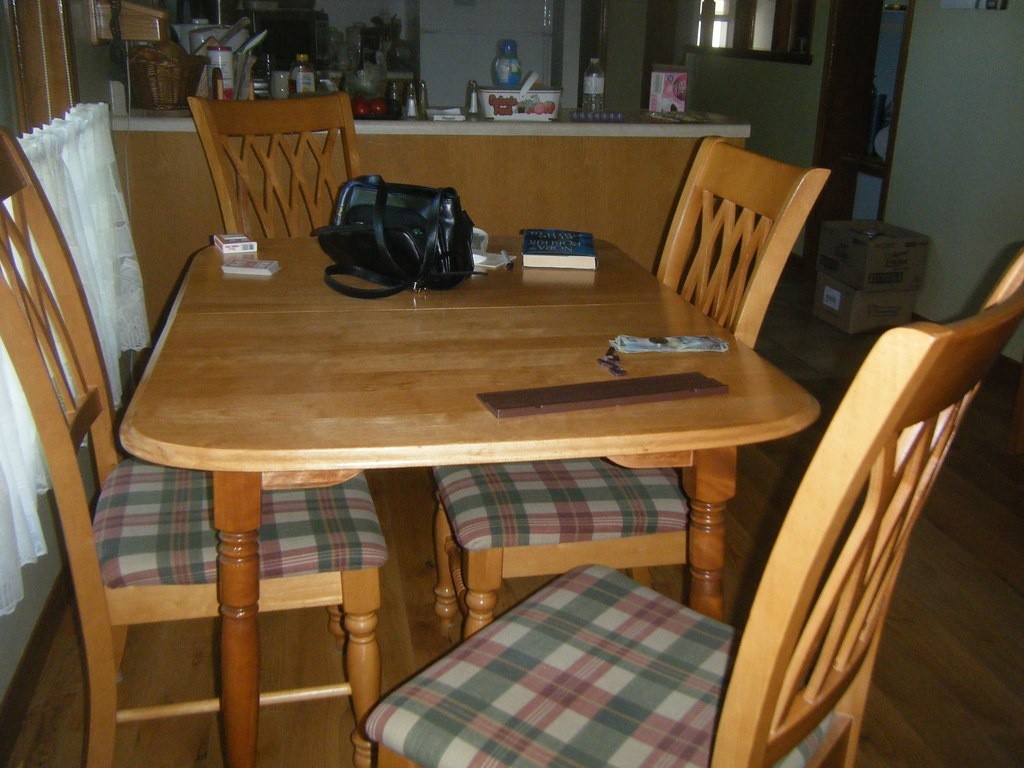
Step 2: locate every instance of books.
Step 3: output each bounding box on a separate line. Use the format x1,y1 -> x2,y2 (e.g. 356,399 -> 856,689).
521,228 -> 598,271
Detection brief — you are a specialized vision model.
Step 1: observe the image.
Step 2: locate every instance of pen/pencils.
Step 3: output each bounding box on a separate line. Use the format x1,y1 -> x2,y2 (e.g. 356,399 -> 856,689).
501,249 -> 514,270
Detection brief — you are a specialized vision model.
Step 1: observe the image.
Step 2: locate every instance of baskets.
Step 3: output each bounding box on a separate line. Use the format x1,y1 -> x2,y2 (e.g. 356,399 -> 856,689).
118,40 -> 211,110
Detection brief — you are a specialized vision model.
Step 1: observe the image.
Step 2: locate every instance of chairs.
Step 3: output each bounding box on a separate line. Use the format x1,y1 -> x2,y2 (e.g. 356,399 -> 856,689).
362,246 -> 1024,768
0,125 -> 389,768
186,93 -> 366,241
429,134 -> 833,642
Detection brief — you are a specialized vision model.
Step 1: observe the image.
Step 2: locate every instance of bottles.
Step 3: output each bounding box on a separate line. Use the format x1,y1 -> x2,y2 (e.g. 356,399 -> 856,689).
388,79 -> 428,121
289,55 -> 319,96
582,58 -> 605,111
465,80 -> 480,122
272,70 -> 290,99
205,45 -> 234,102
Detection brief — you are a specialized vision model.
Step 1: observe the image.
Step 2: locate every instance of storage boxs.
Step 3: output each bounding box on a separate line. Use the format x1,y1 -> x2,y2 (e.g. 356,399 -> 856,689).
816,218 -> 931,293
213,234 -> 257,254
649,71 -> 688,112
813,271 -> 916,335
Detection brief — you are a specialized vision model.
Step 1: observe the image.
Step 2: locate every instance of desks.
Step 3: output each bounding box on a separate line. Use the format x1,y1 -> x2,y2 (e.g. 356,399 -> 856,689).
117,233 -> 822,768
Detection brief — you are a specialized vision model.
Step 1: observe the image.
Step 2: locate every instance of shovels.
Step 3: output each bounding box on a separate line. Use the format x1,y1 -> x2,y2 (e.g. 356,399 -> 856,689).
235,29 -> 268,54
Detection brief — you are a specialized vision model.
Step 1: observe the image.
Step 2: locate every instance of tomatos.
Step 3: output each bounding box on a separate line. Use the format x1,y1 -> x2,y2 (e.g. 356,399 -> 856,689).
350,96 -> 388,117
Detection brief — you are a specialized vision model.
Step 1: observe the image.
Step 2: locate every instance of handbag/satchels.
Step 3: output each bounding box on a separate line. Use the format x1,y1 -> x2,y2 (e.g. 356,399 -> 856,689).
310,175 -> 474,299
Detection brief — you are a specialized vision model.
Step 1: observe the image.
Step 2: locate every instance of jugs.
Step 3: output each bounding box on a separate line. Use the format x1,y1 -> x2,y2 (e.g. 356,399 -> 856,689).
169,17 -> 219,56
